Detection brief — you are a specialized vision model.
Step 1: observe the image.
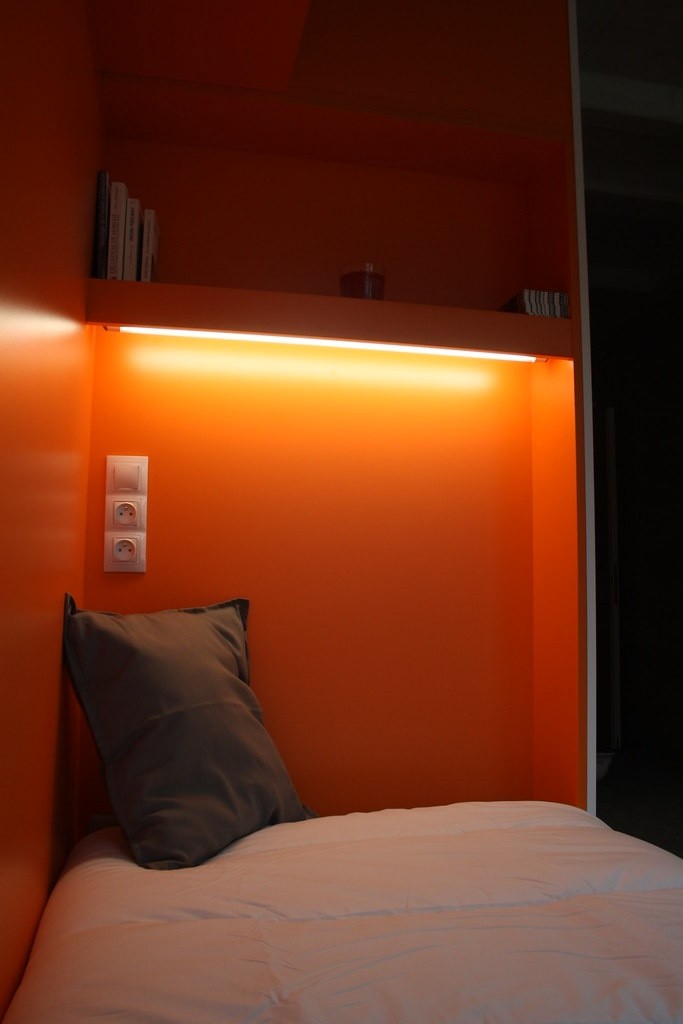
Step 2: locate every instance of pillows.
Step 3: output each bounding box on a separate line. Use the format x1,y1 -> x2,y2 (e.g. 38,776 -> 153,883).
65,592 -> 315,876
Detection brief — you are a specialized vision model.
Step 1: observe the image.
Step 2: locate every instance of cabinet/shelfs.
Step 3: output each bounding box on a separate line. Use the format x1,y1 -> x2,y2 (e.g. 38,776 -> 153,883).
63,2 -> 618,884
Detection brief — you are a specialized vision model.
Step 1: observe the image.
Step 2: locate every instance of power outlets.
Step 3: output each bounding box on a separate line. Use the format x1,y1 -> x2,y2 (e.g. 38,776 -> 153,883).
103,453 -> 152,580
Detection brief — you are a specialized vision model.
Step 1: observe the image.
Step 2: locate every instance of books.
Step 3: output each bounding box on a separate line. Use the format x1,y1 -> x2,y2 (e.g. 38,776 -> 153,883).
140,208 -> 157,282
123,198 -> 140,283
106,181 -> 127,280
495,288 -> 570,320
91,169 -> 110,279
